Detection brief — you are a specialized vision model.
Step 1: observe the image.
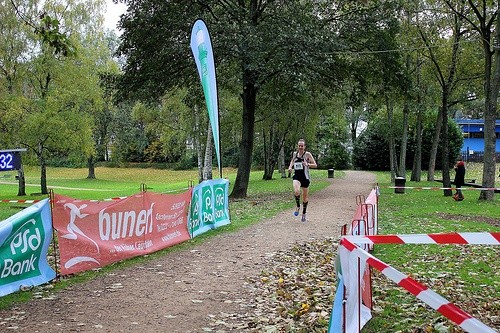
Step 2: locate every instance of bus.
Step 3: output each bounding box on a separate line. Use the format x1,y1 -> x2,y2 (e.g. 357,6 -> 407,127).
452,119 -> 500,162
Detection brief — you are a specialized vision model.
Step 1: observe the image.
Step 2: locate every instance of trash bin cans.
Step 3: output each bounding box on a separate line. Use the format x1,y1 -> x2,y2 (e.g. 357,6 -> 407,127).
395,177 -> 406,194
328,169 -> 334,178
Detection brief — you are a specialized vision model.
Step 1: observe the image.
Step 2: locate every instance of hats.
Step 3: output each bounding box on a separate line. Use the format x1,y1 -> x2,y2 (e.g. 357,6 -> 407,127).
457,161 -> 464,166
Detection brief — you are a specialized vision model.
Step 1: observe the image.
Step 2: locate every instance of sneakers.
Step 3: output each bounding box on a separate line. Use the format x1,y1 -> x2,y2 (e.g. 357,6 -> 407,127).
302,214 -> 307,223
294,208 -> 300,216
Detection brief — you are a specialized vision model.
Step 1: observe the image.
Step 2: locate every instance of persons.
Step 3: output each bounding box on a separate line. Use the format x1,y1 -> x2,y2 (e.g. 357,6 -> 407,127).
454,157 -> 465,201
288,139 -> 317,221
